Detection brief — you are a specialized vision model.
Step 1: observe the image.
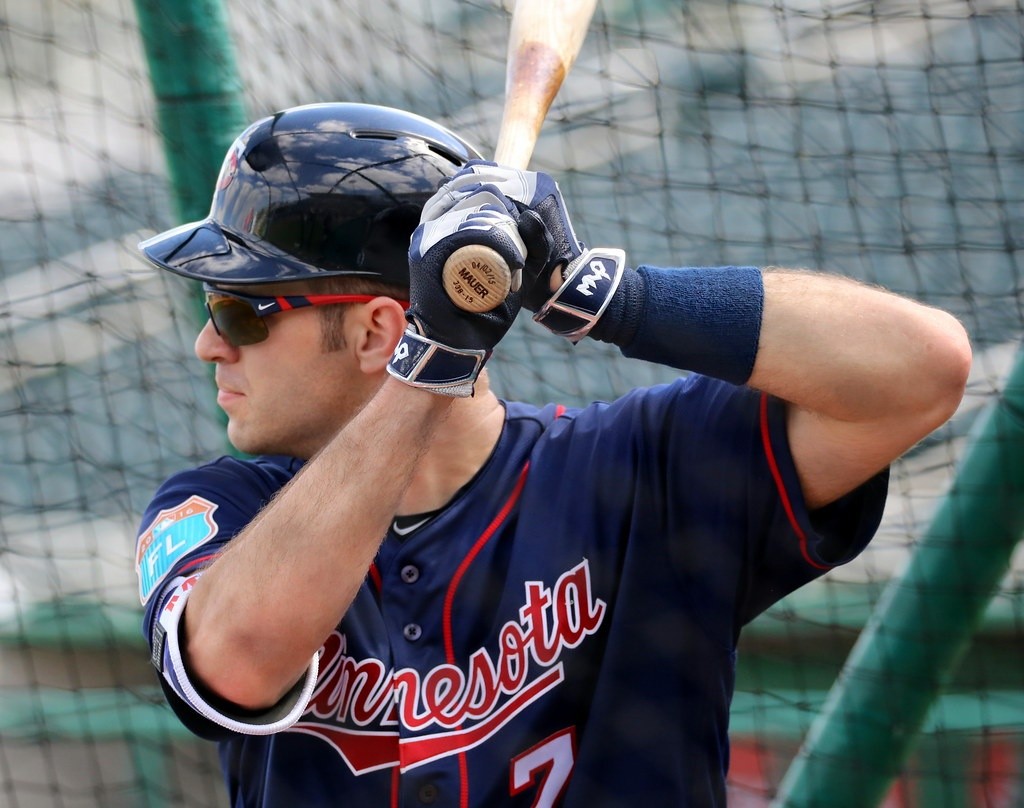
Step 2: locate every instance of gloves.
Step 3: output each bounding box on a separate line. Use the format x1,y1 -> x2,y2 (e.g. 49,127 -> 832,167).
421,157 -> 627,346
386,184 -> 556,400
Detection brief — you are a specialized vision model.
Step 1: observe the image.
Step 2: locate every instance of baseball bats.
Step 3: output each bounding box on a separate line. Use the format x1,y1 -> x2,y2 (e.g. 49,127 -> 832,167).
442,0 -> 598,314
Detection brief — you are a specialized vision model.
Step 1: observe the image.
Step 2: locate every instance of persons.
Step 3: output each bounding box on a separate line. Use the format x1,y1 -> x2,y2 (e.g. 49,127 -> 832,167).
132,103 -> 973,808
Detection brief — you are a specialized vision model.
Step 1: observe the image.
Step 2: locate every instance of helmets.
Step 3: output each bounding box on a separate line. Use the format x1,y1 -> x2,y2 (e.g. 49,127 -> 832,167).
136,103 -> 491,285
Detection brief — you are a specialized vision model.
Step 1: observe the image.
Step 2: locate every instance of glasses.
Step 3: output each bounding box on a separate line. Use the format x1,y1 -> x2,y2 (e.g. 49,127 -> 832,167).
202,281 -> 411,349
